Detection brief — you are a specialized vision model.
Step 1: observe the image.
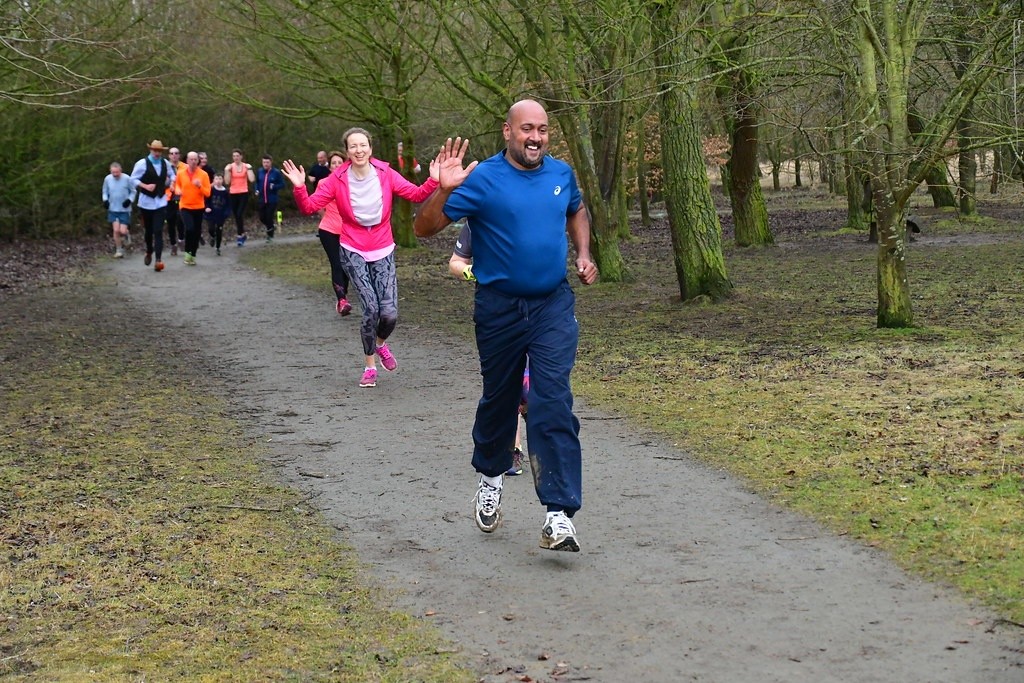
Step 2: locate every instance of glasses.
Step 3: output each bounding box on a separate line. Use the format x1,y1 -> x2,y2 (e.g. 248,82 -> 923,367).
169,151 -> 180,156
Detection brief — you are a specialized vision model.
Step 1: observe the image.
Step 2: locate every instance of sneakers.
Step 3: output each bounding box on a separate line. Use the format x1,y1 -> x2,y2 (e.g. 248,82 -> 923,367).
208,238 -> 216,248
540,513 -> 581,552
266,237 -> 274,243
171,239 -> 184,256
184,252 -> 195,265
143,252 -> 150,265
237,233 -> 248,246
508,445 -> 523,474
154,261 -> 164,271
360,367 -> 378,386
337,292 -> 351,318
472,471 -> 506,532
200,235 -> 205,246
114,247 -> 125,260
216,248 -> 222,256
375,342 -> 397,372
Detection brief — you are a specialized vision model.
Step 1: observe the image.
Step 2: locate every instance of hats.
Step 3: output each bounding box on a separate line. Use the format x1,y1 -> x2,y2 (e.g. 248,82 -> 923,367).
147,139 -> 169,149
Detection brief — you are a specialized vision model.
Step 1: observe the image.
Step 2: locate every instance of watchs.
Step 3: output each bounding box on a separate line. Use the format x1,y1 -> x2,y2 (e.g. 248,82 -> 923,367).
462,265 -> 475,280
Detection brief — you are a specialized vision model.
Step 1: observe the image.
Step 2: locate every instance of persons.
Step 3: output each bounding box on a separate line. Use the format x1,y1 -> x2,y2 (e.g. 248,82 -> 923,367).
450,219 -> 531,476
315,152 -> 352,317
282,127 -> 439,388
307,151 -> 331,193
412,100 -> 597,552
102,140 -> 286,271
